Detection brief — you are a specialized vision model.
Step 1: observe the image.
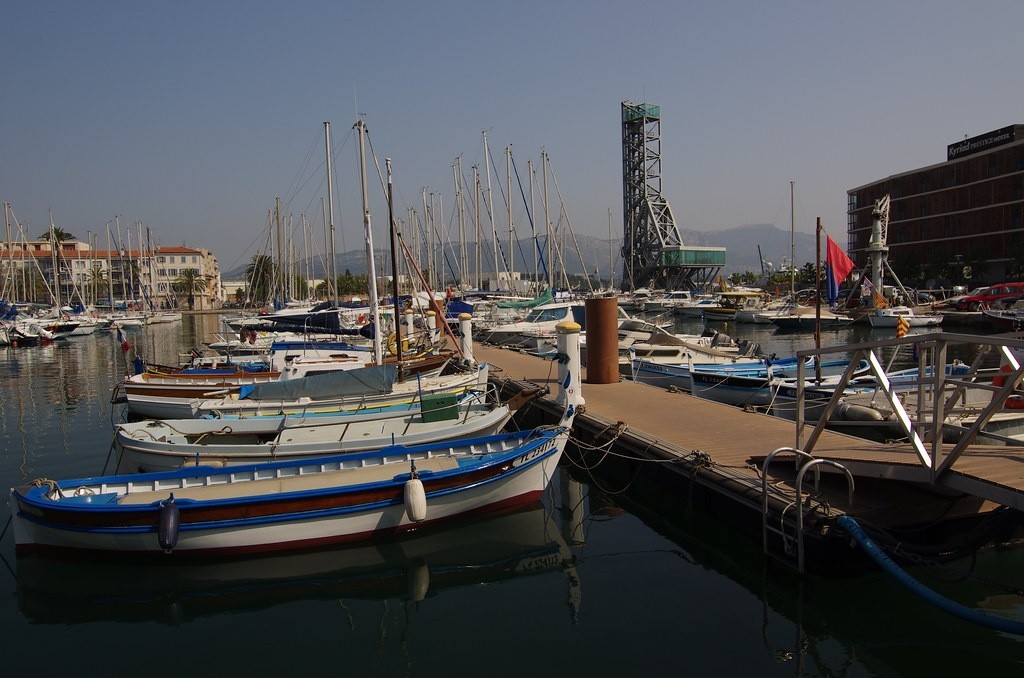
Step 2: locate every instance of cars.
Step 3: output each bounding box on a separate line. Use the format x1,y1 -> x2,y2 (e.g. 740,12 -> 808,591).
896,286 -> 936,303
783,288 -> 821,303
958,283 -> 1024,311
947,286 -> 1010,309
824,288 -> 861,302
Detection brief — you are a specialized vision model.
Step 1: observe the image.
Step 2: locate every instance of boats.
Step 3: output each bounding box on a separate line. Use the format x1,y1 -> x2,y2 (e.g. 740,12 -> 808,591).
112,381 -> 553,471
11,367 -> 576,561
482,292 -> 1024,447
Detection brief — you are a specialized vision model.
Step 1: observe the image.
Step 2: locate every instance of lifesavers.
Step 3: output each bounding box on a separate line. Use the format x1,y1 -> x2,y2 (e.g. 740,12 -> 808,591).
992,363 -> 1024,408
239,329 -> 257,345
387,333 -> 409,355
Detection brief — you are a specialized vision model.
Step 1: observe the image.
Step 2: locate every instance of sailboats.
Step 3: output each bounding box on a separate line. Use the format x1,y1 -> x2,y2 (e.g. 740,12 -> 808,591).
770,181 -> 855,325
0,201 -> 183,345
7,116 -> 490,417
399,131 -> 598,332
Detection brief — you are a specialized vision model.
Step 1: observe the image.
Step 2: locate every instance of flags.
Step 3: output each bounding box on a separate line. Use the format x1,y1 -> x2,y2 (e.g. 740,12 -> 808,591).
875,293 -> 888,309
826,236 -> 854,308
896,315 -> 910,338
864,278 -> 873,290
117,329 -> 130,354
